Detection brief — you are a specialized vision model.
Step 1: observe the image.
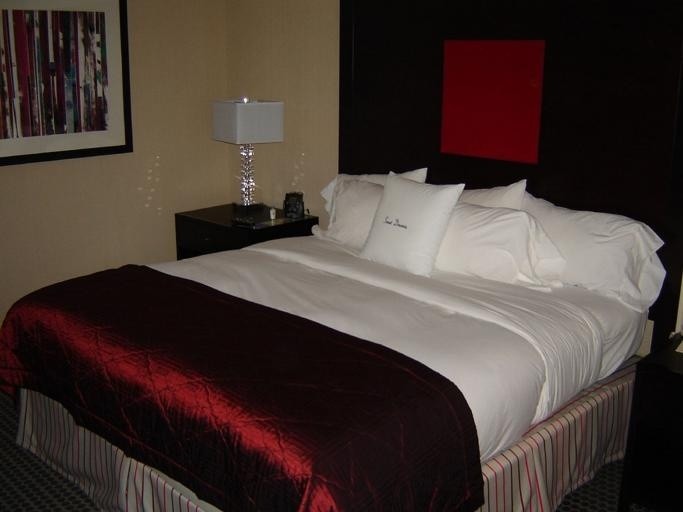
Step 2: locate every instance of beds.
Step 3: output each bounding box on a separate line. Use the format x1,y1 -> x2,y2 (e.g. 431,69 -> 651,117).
0,161 -> 671,512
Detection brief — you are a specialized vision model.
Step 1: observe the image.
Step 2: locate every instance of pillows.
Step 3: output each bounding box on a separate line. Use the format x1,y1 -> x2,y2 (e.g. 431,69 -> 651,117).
319,166 -> 431,225
459,179 -> 527,211
321,180 -> 571,293
520,189 -> 668,313
356,170 -> 468,281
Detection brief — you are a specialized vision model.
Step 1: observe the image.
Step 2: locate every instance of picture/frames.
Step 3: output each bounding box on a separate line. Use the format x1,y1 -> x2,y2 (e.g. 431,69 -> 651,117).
0,0 -> 133,167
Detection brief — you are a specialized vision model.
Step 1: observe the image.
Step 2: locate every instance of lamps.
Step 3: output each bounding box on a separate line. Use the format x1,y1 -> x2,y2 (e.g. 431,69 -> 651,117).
210,95 -> 285,206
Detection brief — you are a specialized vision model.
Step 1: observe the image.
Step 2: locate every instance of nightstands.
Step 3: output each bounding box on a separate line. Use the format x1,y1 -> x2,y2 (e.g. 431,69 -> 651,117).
174,201 -> 321,260
617,335 -> 683,512
